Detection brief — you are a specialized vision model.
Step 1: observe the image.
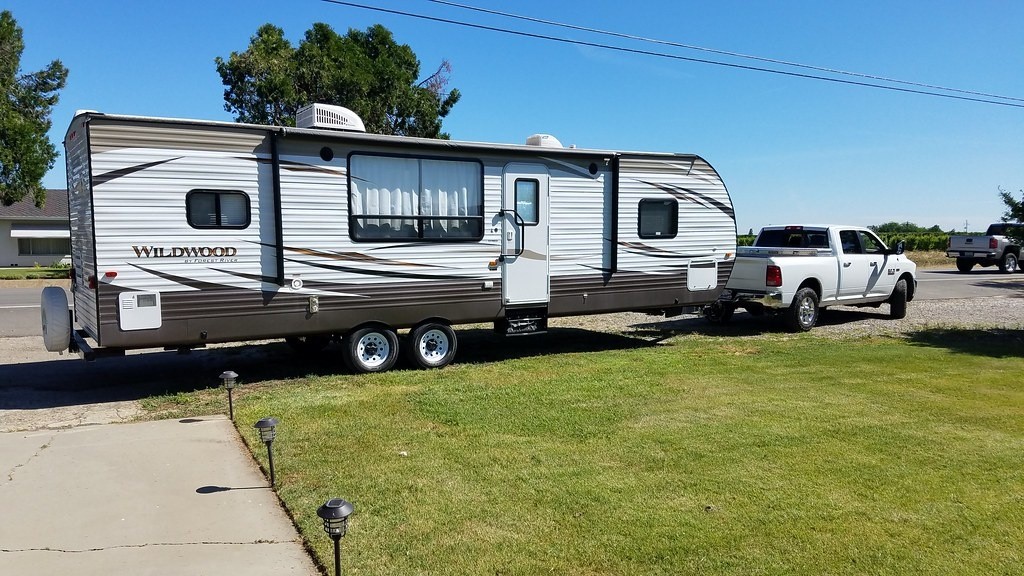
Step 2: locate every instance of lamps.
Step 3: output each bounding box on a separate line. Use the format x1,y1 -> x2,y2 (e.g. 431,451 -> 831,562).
254,418 -> 281,488
317,499 -> 355,576
219,371 -> 240,421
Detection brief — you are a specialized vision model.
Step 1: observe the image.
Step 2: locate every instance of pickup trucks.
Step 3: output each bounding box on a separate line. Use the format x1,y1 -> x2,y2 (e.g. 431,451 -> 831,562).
706,222 -> 918,333
947,221 -> 1024,273
